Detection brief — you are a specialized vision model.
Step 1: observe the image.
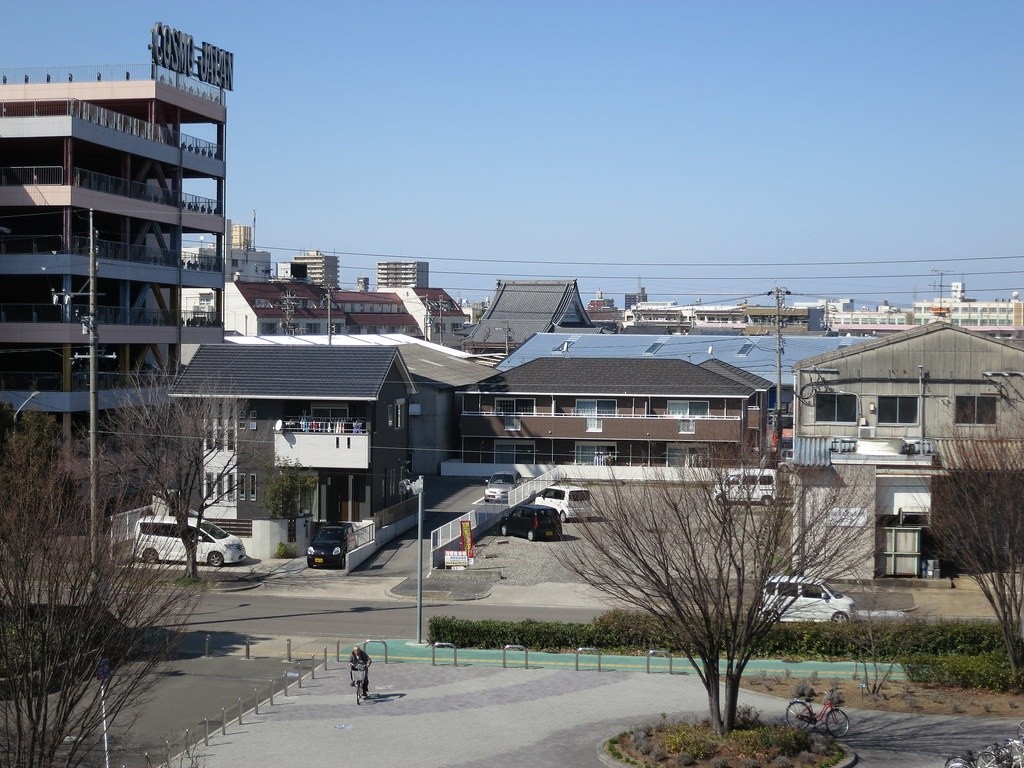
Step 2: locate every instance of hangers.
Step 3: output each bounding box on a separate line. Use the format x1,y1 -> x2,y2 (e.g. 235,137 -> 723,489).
301,416 -> 363,423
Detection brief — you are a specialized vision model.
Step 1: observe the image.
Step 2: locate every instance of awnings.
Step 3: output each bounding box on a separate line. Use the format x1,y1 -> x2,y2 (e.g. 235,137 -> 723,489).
937,439 -> 1024,471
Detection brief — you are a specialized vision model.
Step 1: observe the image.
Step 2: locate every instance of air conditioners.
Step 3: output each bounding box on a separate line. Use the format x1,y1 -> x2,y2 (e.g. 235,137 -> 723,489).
858,426 -> 876,438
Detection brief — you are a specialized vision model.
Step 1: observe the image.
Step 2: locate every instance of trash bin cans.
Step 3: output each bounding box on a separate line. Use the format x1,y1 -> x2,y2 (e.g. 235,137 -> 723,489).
920,559 -> 940,579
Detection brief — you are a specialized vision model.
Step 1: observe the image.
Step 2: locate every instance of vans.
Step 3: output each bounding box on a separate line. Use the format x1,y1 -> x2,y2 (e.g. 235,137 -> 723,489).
132,516 -> 247,567
714,468 -> 777,507
306,523 -> 354,569
761,577 -> 855,624
500,504 -> 562,541
534,485 -> 593,523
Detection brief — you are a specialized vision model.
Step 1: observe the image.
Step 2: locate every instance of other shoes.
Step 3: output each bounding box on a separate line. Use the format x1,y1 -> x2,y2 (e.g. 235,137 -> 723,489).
351,682 -> 354,686
363,691 -> 366,695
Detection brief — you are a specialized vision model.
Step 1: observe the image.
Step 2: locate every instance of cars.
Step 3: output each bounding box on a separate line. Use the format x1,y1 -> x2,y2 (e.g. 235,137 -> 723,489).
777,460 -> 793,472
484,470 -> 524,502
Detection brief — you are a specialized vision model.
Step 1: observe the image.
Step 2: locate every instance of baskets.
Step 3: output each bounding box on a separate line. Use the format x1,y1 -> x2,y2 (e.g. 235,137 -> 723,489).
831,695 -> 844,708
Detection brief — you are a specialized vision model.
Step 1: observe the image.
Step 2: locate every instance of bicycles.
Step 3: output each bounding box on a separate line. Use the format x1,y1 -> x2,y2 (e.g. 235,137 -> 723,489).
785,691 -> 850,739
945,721 -> 1023,768
349,664 -> 369,704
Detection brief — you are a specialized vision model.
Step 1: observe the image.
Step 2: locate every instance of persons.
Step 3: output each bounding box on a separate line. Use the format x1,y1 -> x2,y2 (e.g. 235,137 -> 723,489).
348,645 -> 372,696
